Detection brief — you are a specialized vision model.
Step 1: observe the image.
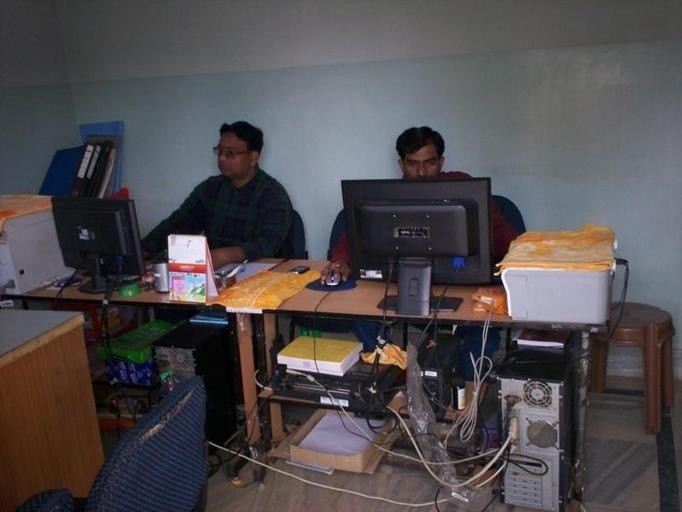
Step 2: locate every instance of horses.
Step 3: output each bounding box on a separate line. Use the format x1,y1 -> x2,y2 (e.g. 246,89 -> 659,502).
289,265 -> 310,274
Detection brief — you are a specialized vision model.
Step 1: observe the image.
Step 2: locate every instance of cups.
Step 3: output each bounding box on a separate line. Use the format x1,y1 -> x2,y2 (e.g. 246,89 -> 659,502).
213,146 -> 250,158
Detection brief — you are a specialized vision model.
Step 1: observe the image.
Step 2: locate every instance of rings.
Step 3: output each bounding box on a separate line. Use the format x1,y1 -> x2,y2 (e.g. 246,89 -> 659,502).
222,258 -> 249,279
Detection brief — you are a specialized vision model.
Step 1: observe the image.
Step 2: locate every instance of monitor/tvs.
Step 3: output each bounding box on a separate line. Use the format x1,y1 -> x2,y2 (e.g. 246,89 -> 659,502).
276,334 -> 364,370
283,352 -> 360,378
188,318 -> 229,326
185,313 -> 231,321
514,327 -> 575,350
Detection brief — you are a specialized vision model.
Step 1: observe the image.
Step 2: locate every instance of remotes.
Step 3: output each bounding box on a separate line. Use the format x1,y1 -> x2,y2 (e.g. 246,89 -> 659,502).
324,272 -> 341,286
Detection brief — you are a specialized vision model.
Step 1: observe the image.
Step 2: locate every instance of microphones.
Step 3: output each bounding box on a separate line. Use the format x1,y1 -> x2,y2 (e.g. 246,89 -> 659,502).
590,303 -> 674,434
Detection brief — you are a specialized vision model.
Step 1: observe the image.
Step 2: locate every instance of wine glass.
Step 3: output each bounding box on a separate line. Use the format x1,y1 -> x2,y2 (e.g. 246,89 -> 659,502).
336,262 -> 343,267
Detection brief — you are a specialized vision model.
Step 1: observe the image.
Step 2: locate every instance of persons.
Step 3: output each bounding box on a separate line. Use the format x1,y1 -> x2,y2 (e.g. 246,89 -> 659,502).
318,121 -> 525,379
140,117 -> 295,447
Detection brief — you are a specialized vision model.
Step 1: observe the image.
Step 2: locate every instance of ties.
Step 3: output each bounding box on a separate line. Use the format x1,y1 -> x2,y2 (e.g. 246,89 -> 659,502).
340,177 -> 491,315
51,196 -> 146,294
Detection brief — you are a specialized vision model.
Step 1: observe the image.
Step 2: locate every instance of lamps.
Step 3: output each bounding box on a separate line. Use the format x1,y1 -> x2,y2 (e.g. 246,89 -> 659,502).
11,375 -> 208,512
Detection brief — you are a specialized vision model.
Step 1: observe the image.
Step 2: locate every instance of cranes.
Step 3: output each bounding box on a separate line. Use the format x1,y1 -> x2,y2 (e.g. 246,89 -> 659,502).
37,140 -> 105,195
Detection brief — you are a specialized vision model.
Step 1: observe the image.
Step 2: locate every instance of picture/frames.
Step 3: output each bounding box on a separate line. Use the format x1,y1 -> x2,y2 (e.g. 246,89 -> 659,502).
238,260 -> 609,512
19,259 -> 281,448
0,309 -> 106,512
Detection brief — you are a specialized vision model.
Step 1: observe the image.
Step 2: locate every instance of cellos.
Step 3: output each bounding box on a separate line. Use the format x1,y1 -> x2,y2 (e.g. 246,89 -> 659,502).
500,225 -> 616,325
0,194 -> 75,295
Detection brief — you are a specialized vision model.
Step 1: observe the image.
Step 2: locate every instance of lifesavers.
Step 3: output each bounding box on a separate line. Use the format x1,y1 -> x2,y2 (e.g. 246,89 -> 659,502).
151,322 -> 237,451
496,328 -> 575,512
